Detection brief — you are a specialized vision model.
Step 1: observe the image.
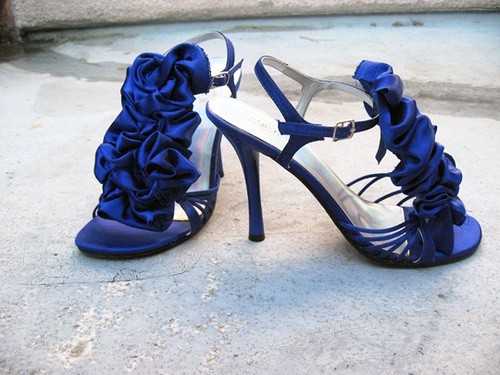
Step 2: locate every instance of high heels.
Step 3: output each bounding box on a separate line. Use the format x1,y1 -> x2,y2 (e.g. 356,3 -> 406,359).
75,28 -> 244,257
205,53 -> 483,268
